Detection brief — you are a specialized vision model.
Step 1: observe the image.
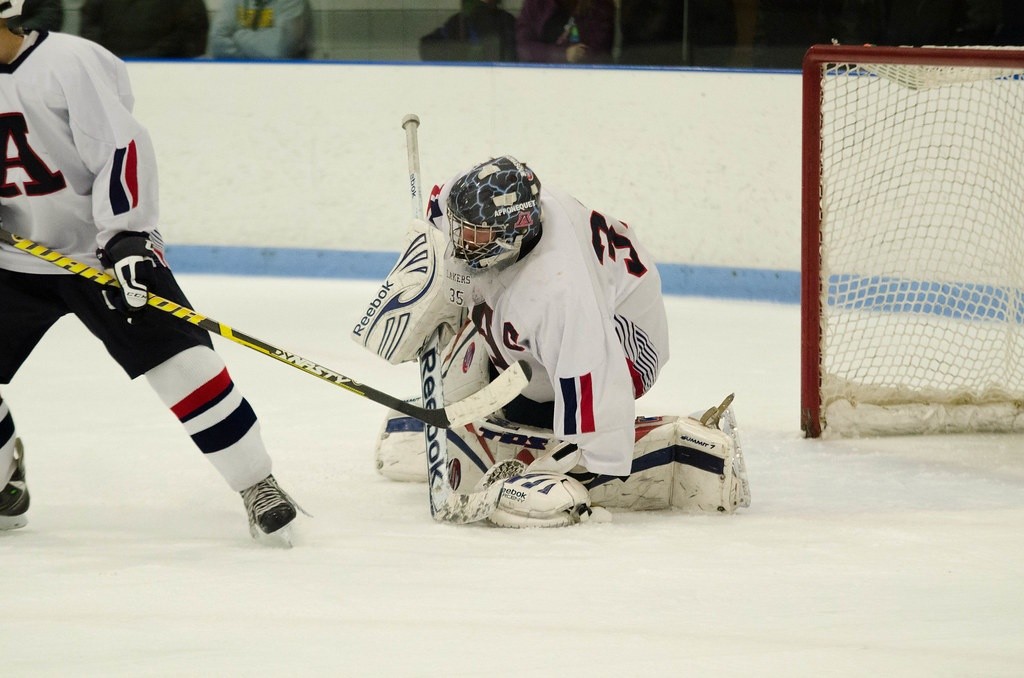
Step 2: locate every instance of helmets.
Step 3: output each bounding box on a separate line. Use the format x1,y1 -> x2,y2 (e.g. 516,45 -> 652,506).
445,157 -> 542,270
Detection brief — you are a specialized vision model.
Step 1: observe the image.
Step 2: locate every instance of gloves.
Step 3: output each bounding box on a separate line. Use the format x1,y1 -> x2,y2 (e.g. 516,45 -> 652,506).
96,233 -> 169,323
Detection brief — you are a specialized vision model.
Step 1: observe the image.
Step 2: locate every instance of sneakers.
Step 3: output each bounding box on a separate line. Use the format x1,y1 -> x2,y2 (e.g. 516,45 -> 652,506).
241,476 -> 301,549
0,437 -> 29,534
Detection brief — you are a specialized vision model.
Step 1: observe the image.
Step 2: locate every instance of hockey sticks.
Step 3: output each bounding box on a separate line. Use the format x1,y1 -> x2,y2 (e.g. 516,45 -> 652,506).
0,226 -> 533,428
400,113 -> 534,524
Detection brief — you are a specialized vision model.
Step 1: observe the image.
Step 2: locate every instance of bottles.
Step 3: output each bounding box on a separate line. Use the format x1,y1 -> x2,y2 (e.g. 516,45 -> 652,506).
569,23 -> 580,46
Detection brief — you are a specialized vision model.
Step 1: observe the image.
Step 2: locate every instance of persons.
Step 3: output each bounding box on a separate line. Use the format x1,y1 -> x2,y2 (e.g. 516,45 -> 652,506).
80,0 -> 210,58
8,0 -> 63,36
212,0 -> 312,58
0,0 -> 314,549
352,156 -> 751,528
622,0 -> 1024,49
420,0 -> 616,65
